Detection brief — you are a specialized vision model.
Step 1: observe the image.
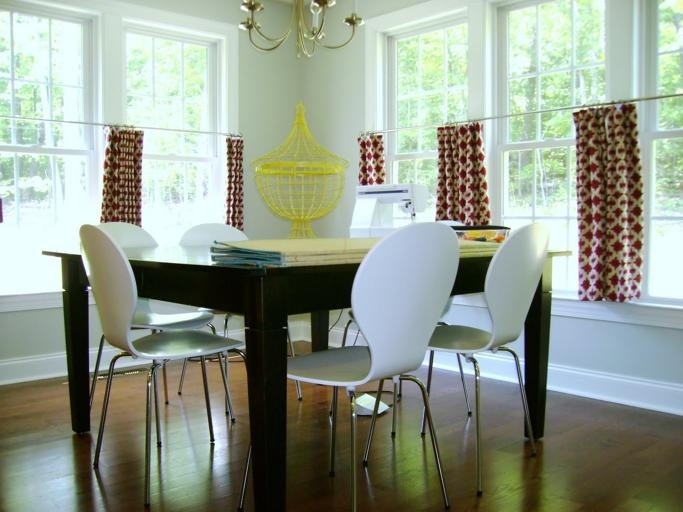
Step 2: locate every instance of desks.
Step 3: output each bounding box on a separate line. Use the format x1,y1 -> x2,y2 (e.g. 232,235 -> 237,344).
40,234 -> 573,511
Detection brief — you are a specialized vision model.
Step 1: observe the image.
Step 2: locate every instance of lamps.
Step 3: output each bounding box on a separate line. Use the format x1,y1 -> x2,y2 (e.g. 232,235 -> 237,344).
237,0 -> 365,60
